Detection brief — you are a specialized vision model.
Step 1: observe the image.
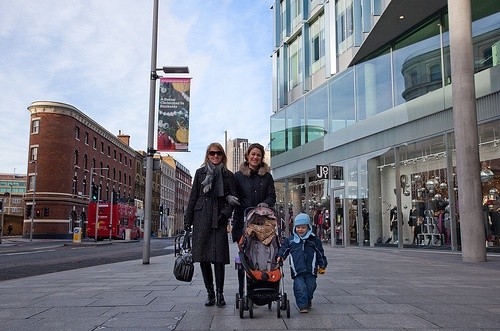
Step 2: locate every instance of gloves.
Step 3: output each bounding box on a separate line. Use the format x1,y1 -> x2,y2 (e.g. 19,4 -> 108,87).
225,195 -> 240,206
318,268 -> 325,274
217,213 -> 227,224
257,202 -> 269,208
184,224 -> 191,232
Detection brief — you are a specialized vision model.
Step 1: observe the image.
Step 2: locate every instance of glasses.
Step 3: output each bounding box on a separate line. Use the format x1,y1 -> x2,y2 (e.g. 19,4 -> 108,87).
209,151 -> 223,156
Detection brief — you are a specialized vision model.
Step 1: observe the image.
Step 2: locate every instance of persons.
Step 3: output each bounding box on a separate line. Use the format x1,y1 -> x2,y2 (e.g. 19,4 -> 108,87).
349,212 -> 356,233
362,210 -> 369,241
280,217 -> 286,236
312,210 -> 319,235
225,143 -> 276,244
390,204 -> 403,245
280,210 -> 329,242
408,204 -> 420,245
275,212 -> 328,313
288,214 -> 293,236
184,143 -> 237,308
7,223 -> 13,236
317,211 -> 327,242
482,196 -> 500,247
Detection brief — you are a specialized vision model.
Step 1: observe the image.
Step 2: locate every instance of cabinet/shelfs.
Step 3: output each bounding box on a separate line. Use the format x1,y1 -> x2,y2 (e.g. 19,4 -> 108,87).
417,217 -> 444,246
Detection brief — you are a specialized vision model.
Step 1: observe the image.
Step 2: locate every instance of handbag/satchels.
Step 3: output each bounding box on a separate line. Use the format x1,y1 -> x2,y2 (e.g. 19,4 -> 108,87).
173,230 -> 194,282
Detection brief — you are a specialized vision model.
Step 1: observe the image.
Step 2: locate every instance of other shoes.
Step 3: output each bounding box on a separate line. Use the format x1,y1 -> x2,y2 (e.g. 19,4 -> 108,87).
300,308 -> 308,312
308,305 -> 311,310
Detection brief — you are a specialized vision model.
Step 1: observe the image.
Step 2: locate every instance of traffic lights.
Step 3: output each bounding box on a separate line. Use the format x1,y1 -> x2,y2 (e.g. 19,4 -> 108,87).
158,205 -> 163,215
36,210 -> 40,216
25,205 -> 31,217
43,207 -> 49,217
166,208 -> 169,216
91,185 -> 98,199
113,192 -> 119,204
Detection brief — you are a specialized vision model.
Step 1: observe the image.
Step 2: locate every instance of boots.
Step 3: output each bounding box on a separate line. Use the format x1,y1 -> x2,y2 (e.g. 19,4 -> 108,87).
205,285 -> 215,306
216,287 -> 226,308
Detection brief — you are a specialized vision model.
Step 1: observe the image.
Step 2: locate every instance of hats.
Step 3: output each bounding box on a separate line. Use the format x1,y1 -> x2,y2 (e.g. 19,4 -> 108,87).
292,213 -> 312,243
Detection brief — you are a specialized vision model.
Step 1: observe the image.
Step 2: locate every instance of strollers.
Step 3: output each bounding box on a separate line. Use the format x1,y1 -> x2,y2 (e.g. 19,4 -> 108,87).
234,205 -> 291,320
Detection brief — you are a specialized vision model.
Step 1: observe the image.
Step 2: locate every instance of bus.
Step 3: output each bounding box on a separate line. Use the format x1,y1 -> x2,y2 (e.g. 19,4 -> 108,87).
86,201 -> 140,240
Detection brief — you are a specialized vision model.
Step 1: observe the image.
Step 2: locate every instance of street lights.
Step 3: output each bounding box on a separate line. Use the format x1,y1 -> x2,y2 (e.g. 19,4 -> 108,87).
88,167 -> 109,203
